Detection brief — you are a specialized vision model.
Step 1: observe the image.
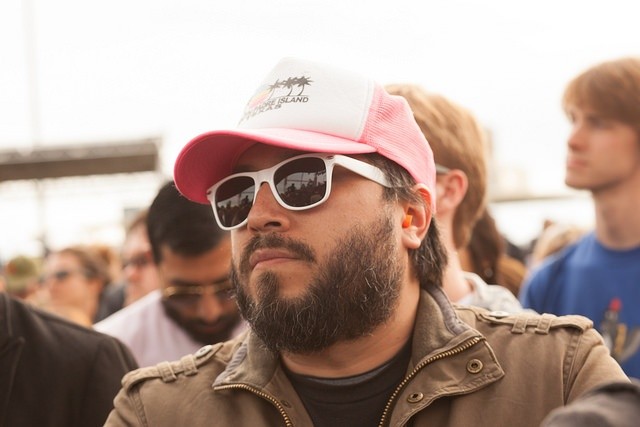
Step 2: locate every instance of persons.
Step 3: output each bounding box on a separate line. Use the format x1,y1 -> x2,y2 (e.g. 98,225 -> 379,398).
0,85 -> 584,427
104,75 -> 640,427
521,57 -> 640,379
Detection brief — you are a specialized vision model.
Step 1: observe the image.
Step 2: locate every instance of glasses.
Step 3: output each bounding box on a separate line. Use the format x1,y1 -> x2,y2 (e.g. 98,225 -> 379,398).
154,267 -> 237,305
205,154 -> 403,231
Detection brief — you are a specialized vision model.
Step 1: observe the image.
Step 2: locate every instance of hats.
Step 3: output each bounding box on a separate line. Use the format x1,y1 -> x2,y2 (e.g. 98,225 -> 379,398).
173,67 -> 436,216
